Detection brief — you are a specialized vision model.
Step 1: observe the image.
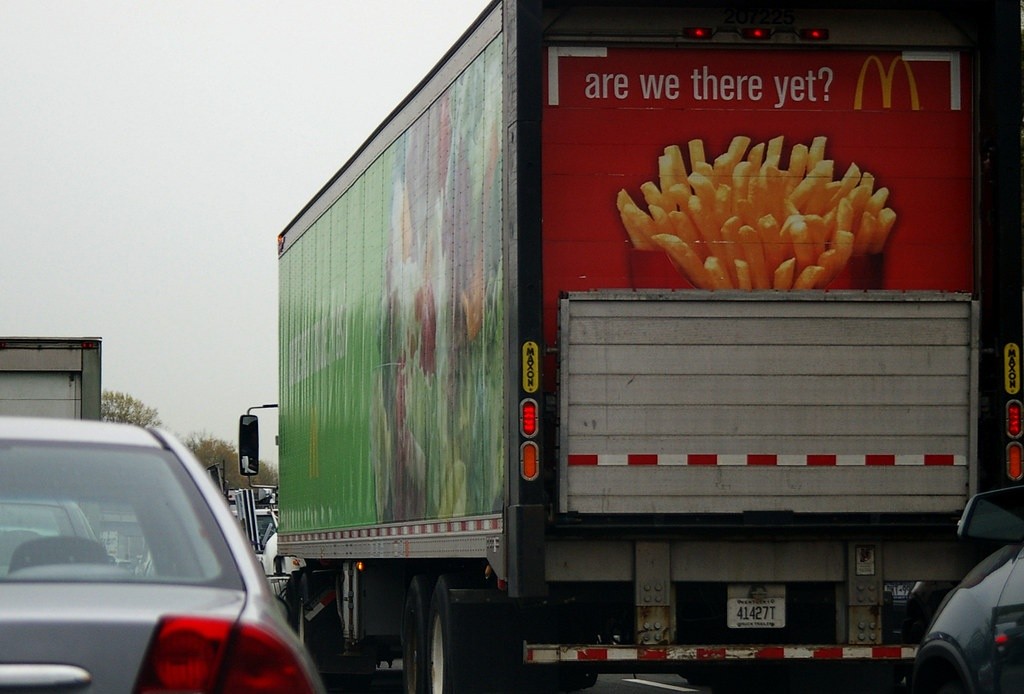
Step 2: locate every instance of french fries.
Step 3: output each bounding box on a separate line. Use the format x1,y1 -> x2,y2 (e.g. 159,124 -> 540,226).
616,136 -> 896,290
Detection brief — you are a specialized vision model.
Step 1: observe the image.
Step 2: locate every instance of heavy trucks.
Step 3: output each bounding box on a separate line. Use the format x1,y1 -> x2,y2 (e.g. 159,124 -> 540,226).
237,0 -> 1024,693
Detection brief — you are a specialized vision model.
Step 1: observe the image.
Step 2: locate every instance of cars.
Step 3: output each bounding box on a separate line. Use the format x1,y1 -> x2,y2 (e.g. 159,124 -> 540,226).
1,500 -> 99,574
0,415 -> 327,693
909,483 -> 1024,694
